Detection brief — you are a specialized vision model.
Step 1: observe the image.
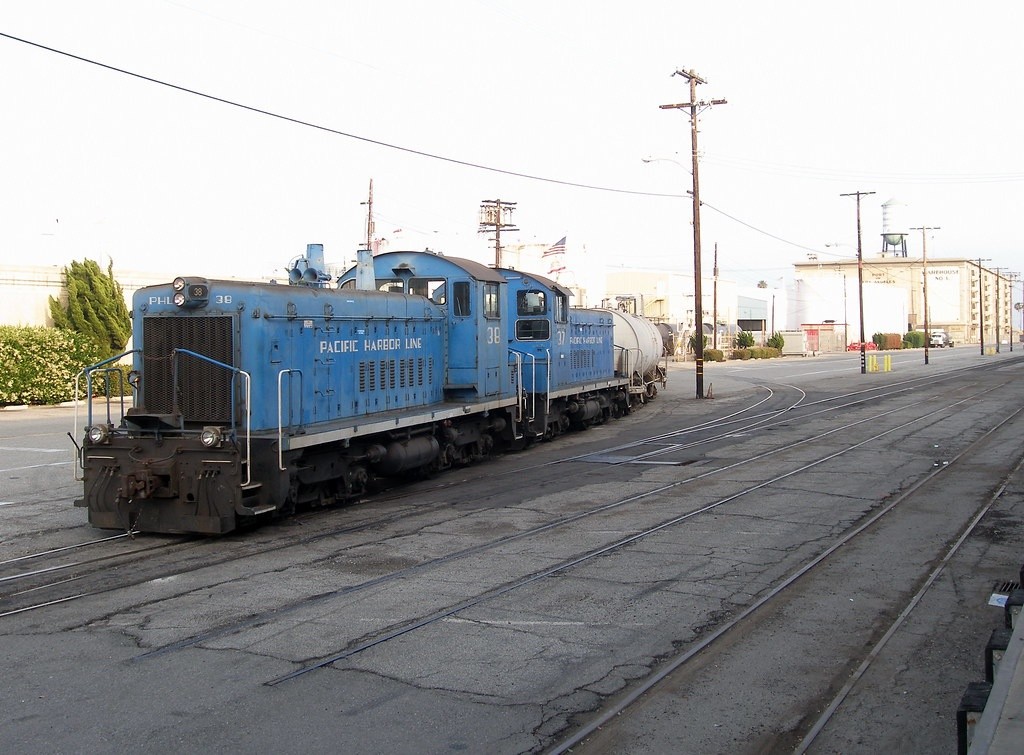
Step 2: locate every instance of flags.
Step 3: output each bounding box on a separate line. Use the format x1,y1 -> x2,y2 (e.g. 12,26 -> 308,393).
542,236 -> 567,258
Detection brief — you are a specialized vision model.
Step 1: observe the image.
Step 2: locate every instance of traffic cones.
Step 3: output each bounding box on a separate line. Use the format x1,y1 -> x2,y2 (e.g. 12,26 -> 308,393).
705,382 -> 715,400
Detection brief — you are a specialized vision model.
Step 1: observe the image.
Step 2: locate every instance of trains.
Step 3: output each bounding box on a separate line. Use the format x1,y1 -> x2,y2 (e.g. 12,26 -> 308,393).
66,243 -> 669,536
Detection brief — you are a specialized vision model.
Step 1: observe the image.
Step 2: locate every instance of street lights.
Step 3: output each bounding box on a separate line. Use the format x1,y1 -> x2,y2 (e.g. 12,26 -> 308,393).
825,242 -> 867,374
642,157 -> 705,400
